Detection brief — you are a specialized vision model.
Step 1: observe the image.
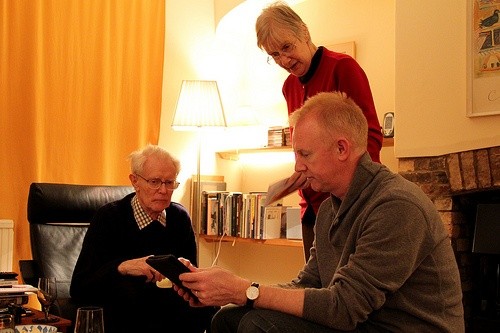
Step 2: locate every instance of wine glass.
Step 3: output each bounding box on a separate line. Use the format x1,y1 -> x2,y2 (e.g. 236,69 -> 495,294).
37,277 -> 60,323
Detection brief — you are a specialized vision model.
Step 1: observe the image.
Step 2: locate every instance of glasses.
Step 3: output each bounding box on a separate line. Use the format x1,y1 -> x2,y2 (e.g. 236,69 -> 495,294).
267,38 -> 297,65
135,173 -> 180,190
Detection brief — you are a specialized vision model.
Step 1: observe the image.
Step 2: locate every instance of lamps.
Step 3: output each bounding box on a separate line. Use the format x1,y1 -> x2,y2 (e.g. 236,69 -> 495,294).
170,79 -> 227,269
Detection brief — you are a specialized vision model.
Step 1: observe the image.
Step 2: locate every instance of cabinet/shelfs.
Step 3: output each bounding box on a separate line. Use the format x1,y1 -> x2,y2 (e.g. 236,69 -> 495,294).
194,139 -> 394,286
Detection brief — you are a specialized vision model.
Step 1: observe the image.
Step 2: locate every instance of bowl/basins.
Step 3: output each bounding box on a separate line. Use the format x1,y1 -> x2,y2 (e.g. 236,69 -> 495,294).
0,325 -> 57,333
0,272 -> 19,279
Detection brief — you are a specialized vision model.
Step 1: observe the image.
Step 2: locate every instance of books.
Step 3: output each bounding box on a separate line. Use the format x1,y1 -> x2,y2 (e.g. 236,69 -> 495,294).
190,175 -> 303,240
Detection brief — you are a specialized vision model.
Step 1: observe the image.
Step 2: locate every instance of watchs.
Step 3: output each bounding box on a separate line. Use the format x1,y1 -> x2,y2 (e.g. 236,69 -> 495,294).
245,282 -> 261,309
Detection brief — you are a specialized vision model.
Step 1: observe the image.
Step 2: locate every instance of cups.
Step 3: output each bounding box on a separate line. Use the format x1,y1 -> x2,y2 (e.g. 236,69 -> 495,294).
0,314 -> 14,333
74,307 -> 104,333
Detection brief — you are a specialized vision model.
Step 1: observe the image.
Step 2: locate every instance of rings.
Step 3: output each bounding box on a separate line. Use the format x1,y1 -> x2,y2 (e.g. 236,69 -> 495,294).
196,290 -> 200,298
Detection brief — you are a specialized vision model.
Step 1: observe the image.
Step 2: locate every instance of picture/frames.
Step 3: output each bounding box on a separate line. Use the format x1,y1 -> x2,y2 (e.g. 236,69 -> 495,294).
466,0 -> 500,118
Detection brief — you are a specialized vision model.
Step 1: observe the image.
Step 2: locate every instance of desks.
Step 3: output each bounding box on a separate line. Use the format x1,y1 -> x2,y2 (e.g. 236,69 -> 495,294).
0,305 -> 72,333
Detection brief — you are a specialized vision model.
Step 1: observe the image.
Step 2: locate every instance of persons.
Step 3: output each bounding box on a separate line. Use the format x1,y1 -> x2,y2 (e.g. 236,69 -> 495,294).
256,3 -> 383,264
173,91 -> 464,333
71,146 -> 222,333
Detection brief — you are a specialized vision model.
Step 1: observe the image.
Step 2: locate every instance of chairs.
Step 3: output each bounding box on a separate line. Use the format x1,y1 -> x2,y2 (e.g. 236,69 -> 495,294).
27,183 -> 136,321
